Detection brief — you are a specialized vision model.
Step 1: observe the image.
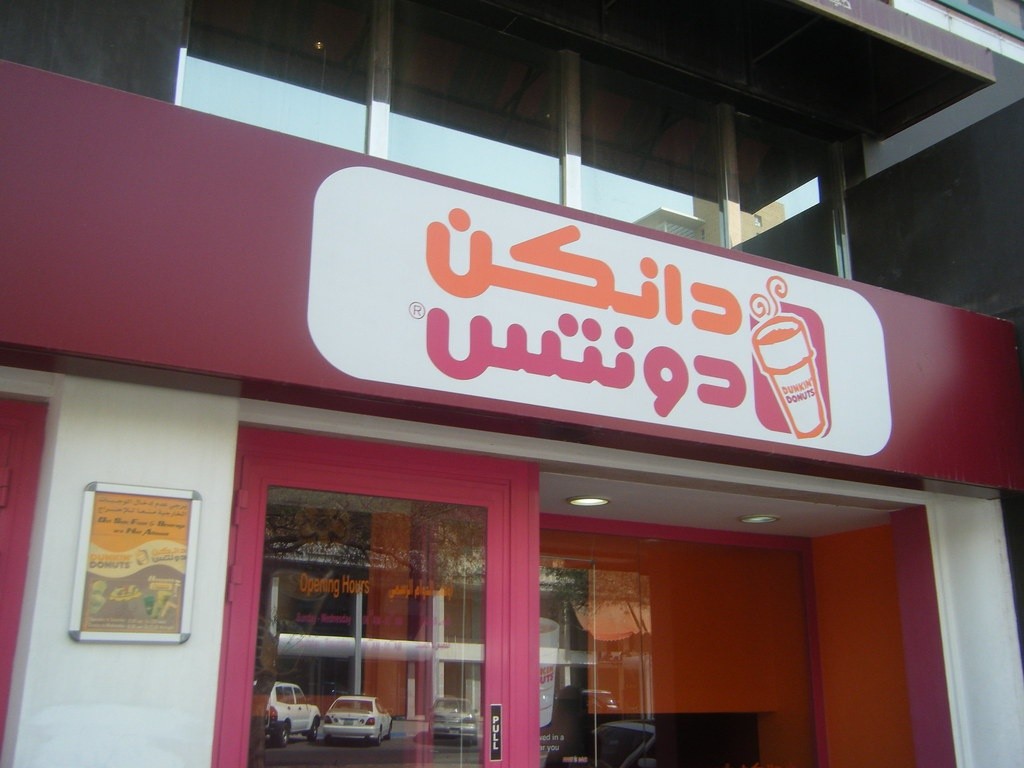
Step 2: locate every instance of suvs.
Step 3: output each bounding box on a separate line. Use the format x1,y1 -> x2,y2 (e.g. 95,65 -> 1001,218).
252,682 -> 322,747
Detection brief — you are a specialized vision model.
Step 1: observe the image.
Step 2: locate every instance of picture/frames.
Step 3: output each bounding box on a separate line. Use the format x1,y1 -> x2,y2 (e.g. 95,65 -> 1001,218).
67,481 -> 203,644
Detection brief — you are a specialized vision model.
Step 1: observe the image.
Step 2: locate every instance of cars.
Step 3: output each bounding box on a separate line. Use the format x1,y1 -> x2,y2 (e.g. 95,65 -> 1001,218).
428,696 -> 480,747
592,720 -> 657,768
320,696 -> 393,745
